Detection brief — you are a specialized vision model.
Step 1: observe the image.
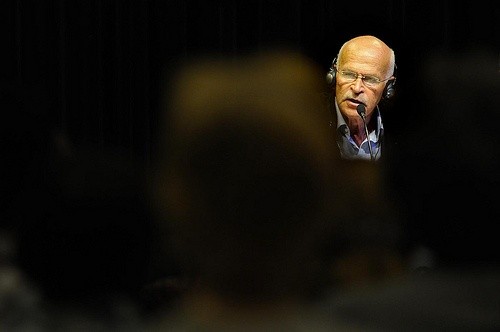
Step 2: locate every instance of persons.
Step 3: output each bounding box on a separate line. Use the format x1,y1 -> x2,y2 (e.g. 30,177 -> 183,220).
321,35 -> 397,165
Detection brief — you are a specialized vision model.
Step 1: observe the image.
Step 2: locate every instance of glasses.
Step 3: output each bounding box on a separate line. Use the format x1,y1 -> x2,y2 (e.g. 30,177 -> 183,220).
336,70 -> 390,86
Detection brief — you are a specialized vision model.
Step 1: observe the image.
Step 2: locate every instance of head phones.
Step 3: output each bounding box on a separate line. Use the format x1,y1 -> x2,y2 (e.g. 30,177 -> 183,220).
326,57 -> 396,101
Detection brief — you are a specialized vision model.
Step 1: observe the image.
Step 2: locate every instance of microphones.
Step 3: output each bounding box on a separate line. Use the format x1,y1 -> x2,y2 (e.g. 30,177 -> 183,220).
357,104 -> 373,160
